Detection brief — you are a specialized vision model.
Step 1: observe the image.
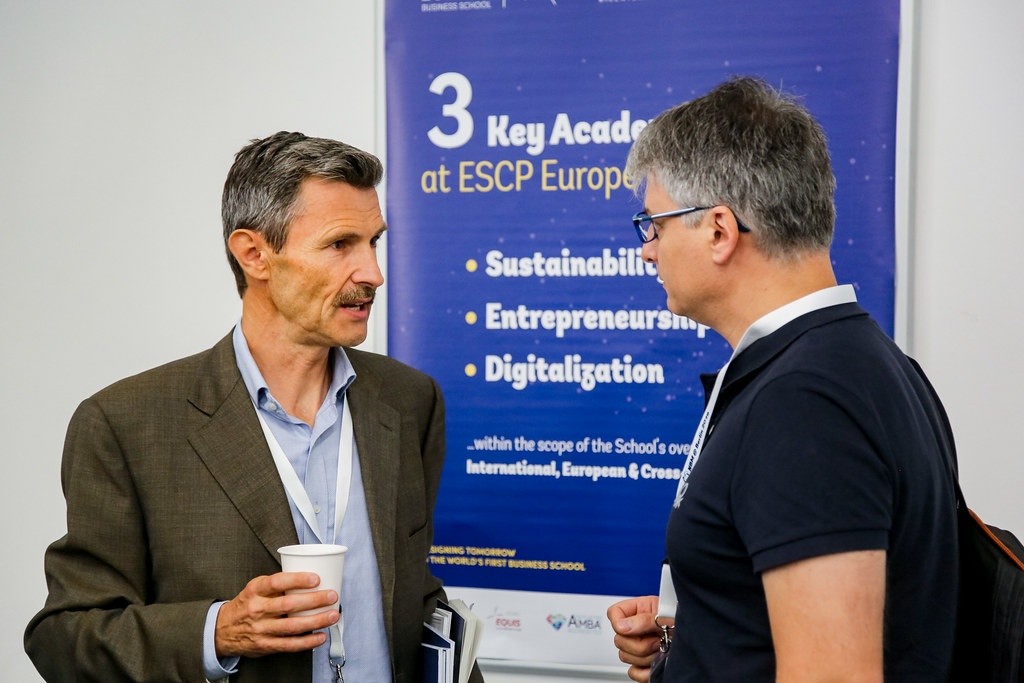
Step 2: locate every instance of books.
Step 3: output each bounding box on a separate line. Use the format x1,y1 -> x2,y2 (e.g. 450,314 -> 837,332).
419,596 -> 485,683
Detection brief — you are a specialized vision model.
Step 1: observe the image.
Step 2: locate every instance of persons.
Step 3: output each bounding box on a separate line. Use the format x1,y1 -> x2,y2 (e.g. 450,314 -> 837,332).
22,129 -> 490,683
606,72 -> 961,683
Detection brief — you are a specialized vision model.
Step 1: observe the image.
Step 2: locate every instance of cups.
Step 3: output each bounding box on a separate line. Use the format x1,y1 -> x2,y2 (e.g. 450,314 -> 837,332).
277,544 -> 348,627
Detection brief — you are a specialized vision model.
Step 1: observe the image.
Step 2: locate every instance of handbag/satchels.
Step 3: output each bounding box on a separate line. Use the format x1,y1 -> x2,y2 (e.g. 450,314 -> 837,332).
909,356 -> 1024,683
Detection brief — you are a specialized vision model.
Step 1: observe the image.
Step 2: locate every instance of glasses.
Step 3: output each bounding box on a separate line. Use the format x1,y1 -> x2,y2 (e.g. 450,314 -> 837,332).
632,205 -> 750,243
